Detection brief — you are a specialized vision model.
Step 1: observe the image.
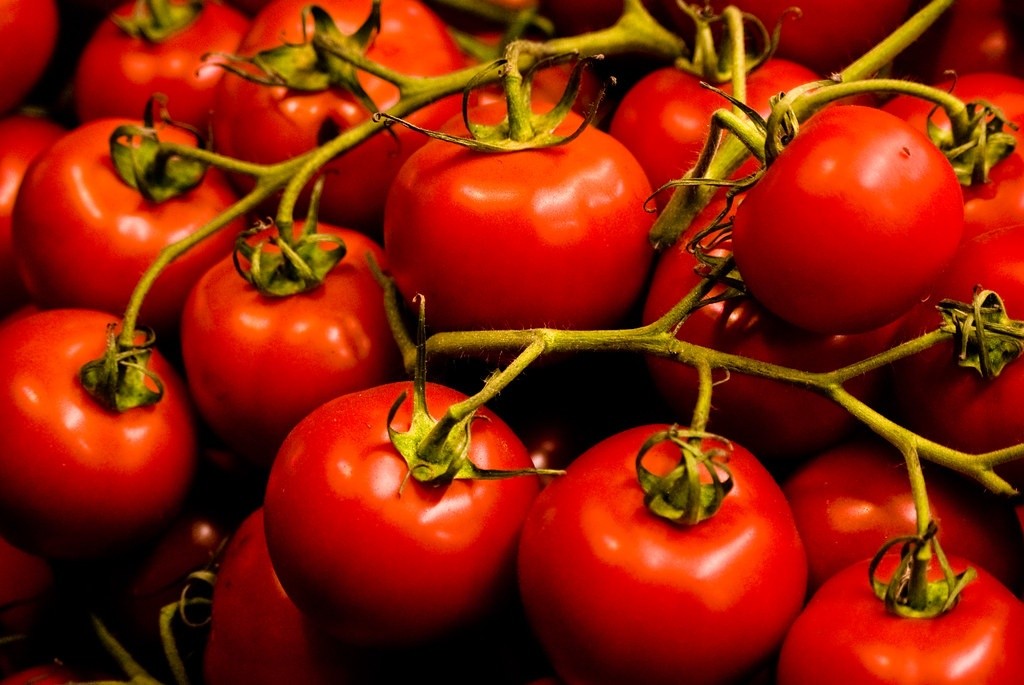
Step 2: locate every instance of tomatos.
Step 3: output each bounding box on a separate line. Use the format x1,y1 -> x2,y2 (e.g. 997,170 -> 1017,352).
0,0 -> 1024,685
